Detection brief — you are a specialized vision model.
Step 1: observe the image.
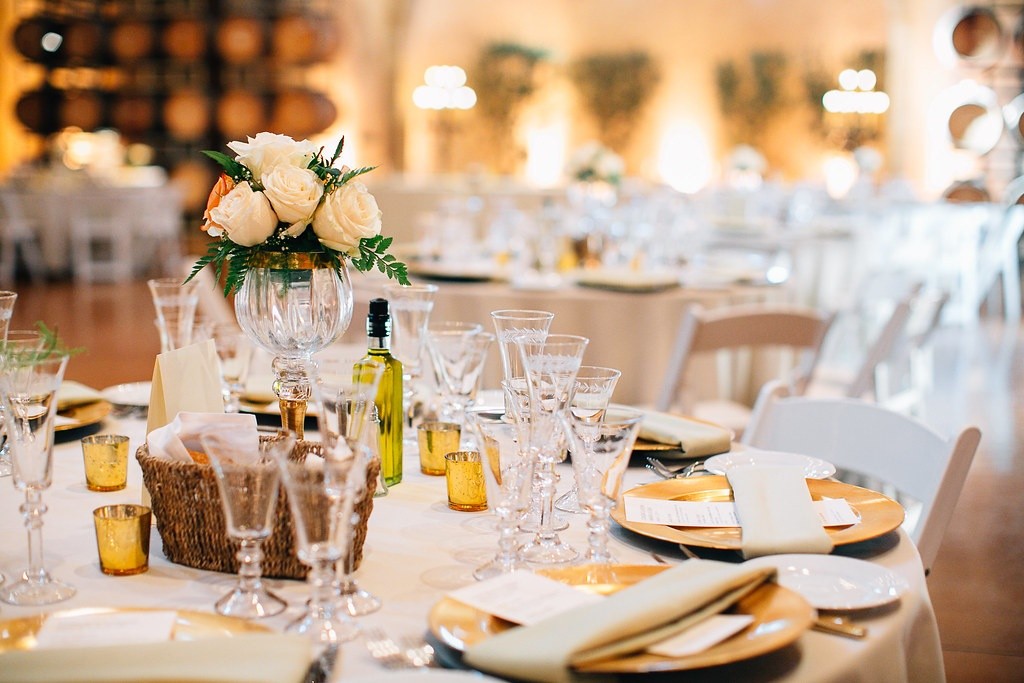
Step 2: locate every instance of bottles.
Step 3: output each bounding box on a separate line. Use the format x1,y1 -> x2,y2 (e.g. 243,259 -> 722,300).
353,300 -> 404,487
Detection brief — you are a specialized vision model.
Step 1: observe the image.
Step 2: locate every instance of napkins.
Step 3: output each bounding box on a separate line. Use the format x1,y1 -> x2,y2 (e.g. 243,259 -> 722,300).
465,558 -> 776,683
604,408 -> 730,459
726,468 -> 832,560
0,643 -> 338,683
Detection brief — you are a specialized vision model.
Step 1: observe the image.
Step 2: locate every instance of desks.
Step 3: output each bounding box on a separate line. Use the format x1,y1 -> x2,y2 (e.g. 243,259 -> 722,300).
0,167 -> 174,288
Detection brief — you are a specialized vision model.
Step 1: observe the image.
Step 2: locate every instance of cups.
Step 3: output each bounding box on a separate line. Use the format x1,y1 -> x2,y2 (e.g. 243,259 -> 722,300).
417,422 -> 460,476
80,432 -> 130,491
444,452 -> 489,513
92,504 -> 153,577
147,276 -> 249,414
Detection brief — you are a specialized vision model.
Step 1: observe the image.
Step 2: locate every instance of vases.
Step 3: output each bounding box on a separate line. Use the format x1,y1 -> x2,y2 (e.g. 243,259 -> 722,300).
232,250 -> 354,438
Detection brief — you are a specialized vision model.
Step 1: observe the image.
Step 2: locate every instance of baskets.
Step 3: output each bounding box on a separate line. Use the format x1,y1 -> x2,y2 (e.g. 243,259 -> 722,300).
136,432 -> 382,579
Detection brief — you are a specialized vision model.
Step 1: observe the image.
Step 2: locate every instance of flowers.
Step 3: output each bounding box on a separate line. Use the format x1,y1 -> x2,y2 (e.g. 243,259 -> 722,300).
181,133 -> 411,298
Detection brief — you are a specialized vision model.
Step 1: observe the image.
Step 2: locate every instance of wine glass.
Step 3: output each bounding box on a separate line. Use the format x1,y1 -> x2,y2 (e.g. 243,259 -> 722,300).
0,290 -> 77,607
385,282 -> 642,591
201,422 -> 379,645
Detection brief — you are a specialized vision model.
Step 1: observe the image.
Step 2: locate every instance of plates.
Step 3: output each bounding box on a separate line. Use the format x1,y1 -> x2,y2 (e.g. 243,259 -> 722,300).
100,381 -> 154,408
560,407 -> 907,551
427,563 -> 819,678
53,399 -> 111,432
744,554 -> 907,613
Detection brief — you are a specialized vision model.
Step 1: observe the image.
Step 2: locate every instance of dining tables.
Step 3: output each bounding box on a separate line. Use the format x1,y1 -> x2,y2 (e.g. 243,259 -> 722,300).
0,391 -> 943,683
318,239 -> 800,412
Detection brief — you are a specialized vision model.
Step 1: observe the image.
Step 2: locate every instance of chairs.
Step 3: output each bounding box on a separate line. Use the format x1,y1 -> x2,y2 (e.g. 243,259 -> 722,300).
645,208 -> 1024,582
